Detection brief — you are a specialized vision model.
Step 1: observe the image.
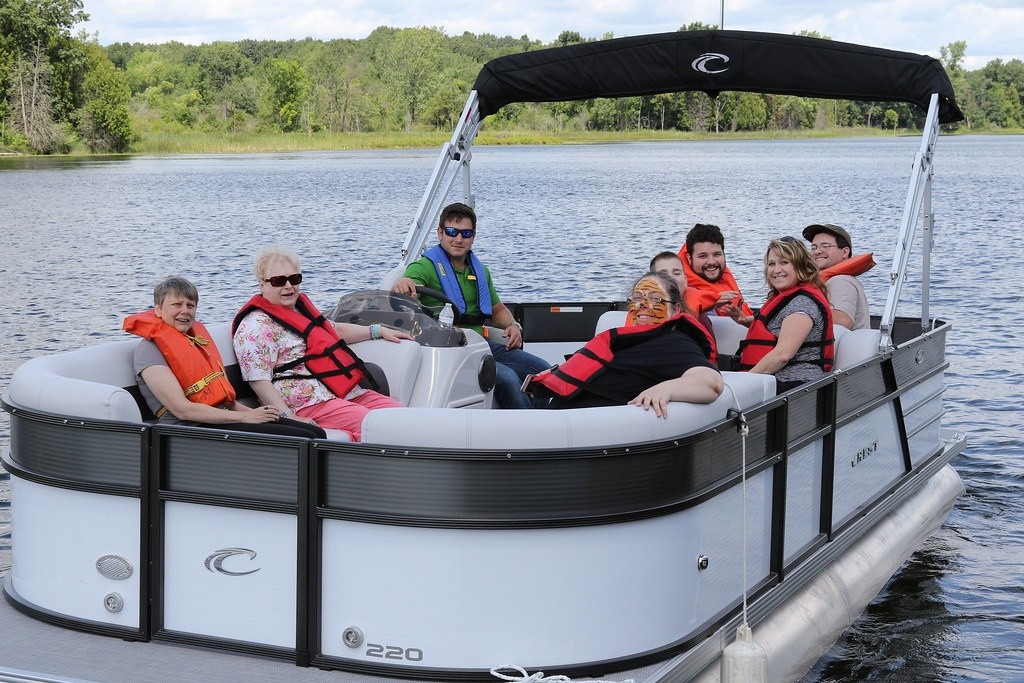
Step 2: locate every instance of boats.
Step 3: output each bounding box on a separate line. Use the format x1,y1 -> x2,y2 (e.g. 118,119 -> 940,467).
0,26 -> 968,683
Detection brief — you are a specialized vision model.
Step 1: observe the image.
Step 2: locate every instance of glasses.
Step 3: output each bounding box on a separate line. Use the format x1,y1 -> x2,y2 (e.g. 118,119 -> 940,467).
626,294 -> 676,307
262,273 -> 302,288
441,226 -> 474,239
806,243 -> 838,251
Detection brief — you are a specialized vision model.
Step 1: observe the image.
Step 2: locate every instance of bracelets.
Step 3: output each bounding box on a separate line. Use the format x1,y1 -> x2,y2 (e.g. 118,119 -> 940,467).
369,325 -> 372,339
373,324 -> 378,339
513,323 -> 522,331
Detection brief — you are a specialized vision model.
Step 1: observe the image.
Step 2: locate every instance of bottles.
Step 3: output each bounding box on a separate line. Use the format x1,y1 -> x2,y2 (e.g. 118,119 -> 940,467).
438,303 -> 454,328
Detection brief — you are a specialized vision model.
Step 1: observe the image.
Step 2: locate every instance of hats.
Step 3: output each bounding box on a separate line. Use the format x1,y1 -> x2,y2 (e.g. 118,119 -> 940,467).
802,224 -> 852,249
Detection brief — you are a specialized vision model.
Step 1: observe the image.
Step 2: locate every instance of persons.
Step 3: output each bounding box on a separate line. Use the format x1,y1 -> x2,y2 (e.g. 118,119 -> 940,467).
623,251 -> 718,350
717,236 -> 834,393
133,277 -> 328,439
802,224 -> 870,331
678,223 -> 753,316
533,271 -> 722,418
232,245 -> 415,442
390,203 -> 552,409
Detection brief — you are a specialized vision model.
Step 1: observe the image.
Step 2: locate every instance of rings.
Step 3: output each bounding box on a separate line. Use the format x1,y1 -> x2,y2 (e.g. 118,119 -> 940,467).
267,410 -> 270,413
